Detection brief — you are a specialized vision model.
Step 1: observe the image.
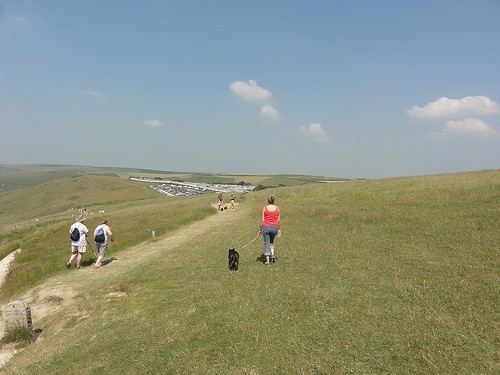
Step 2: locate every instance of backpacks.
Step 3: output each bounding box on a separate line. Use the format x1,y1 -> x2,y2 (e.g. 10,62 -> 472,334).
70,223 -> 84,241
94,225 -> 106,243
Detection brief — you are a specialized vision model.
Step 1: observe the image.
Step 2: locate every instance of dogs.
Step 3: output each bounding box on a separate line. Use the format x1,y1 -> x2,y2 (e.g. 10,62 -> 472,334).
228,249 -> 240,272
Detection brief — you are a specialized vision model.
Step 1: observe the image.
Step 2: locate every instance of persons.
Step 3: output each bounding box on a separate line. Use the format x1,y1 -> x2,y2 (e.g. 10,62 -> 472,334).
66,215 -> 90,270
84,208 -> 86,213
217,192 -> 236,209
258,195 -> 281,264
94,219 -> 114,269
99,209 -> 105,215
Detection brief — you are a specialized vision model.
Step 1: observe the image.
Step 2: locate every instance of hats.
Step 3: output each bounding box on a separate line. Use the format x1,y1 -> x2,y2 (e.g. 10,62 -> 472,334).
76,215 -> 85,223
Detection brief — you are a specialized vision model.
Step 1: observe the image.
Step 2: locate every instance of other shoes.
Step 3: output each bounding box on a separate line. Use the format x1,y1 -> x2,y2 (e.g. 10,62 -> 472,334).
95,266 -> 98,269
66,262 -> 72,269
77,267 -> 82,269
